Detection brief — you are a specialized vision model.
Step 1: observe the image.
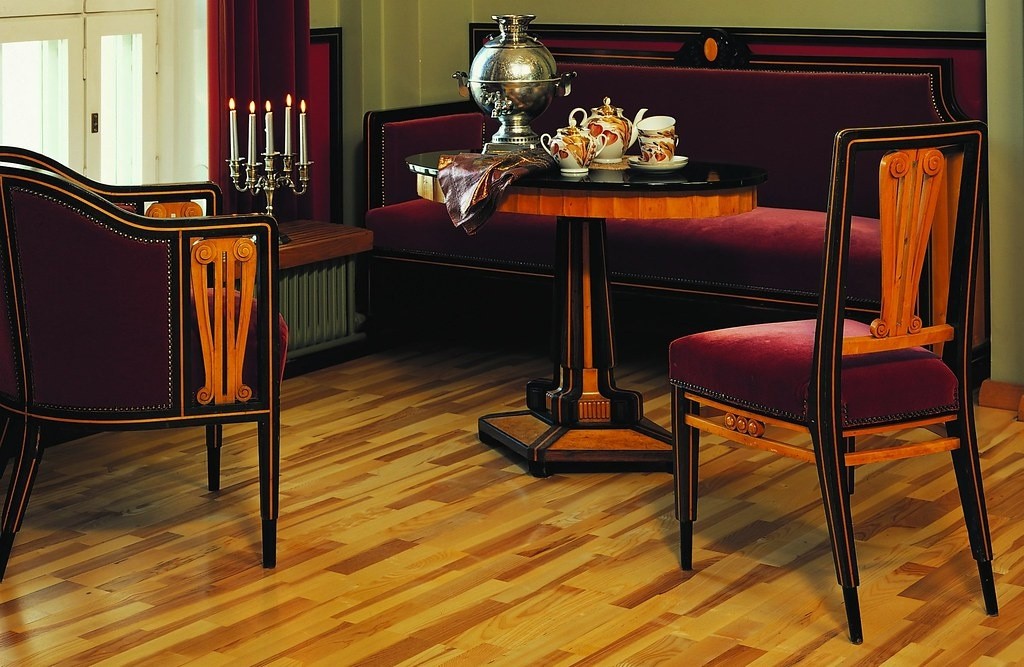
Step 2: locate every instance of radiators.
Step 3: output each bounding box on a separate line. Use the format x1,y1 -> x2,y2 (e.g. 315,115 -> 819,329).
221,252 -> 367,361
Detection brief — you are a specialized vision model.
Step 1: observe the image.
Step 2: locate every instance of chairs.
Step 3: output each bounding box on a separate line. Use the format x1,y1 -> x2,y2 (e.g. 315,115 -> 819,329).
667,121 -> 1001,646
0,143 -> 286,591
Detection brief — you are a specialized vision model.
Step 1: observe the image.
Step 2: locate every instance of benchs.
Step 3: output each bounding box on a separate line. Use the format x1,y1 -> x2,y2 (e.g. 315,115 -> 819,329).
354,21 -> 991,412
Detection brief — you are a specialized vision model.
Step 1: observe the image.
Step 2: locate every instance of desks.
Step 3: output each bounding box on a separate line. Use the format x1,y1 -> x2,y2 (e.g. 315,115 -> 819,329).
405,150 -> 768,477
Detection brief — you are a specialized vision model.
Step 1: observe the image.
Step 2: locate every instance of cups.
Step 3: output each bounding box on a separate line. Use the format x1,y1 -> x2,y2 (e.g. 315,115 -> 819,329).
637,116 -> 676,137
638,136 -> 676,162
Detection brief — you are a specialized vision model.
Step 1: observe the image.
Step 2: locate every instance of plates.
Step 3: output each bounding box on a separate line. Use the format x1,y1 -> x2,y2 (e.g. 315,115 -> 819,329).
629,155 -> 688,164
628,161 -> 686,173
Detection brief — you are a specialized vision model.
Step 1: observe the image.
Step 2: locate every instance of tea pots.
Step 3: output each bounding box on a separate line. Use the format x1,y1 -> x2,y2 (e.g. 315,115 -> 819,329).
569,97 -> 648,164
540,118 -> 607,177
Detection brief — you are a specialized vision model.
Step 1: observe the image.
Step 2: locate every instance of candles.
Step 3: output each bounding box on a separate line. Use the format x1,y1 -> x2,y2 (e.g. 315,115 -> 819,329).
228,97 -> 239,161
285,93 -> 291,153
299,100 -> 308,163
247,100 -> 257,164
264,100 -> 273,154
